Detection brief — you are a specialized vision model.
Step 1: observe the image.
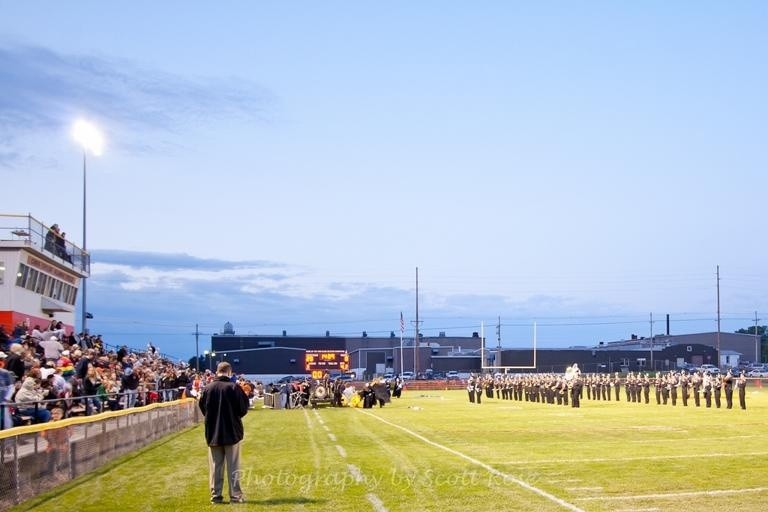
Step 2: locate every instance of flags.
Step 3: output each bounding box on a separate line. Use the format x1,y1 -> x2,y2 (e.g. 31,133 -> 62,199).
399,313 -> 404,332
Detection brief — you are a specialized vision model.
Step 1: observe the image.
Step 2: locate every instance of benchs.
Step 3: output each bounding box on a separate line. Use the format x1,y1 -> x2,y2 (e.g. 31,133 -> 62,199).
20,415 -> 32,425
71,401 -> 124,416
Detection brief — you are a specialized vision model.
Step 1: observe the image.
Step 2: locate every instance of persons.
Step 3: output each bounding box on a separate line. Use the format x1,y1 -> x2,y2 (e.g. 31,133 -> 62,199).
466,363 -> 746,410
43,224 -> 72,263
0,318 -> 404,504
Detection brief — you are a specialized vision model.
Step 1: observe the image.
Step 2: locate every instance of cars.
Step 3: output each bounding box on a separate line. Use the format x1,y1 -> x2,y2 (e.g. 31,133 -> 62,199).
384,370 -> 459,380
338,376 -> 352,382
747,369 -> 768,378
682,363 -> 720,376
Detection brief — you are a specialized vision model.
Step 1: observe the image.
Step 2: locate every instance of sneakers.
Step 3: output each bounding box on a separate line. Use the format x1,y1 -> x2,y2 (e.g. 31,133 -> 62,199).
209,494 -> 247,506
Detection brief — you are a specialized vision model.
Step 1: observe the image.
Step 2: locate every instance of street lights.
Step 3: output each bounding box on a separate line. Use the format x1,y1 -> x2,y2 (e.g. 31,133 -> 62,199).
73,119 -> 106,335
204,350 -> 216,370
